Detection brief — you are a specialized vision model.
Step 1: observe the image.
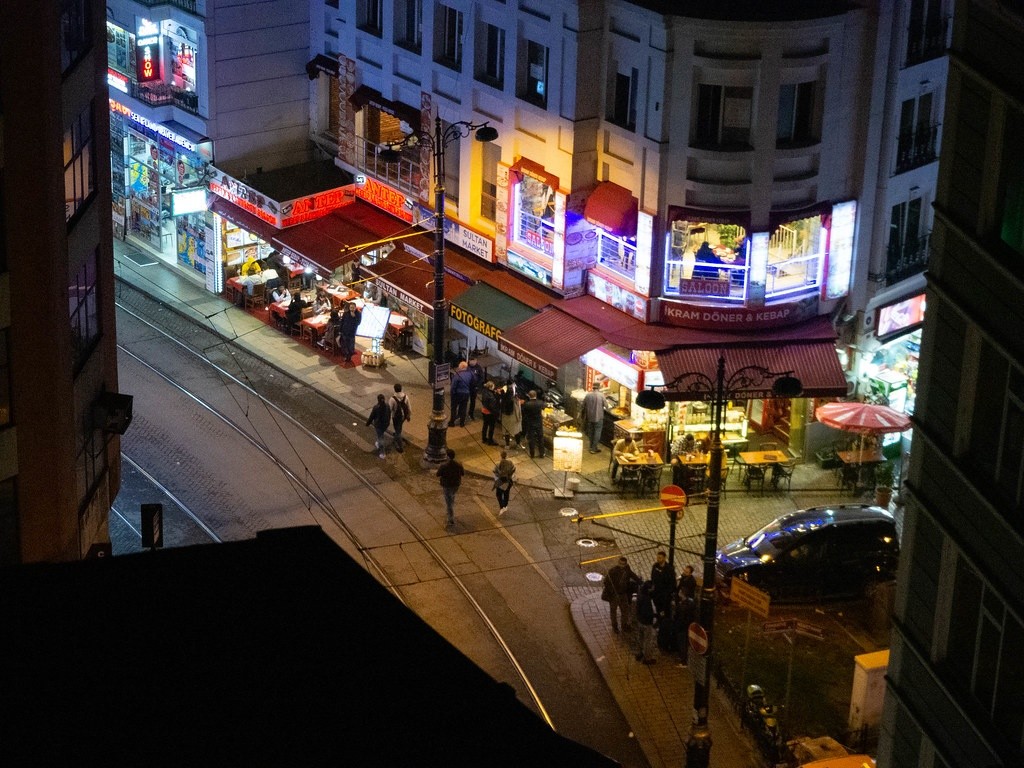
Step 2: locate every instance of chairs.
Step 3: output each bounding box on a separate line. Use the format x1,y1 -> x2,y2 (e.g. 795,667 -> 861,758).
325,325 -> 341,354
244,284 -> 264,308
741,464 -> 768,496
291,307 -> 314,344
730,442 -> 748,481
607,438 -> 618,470
223,265 -> 237,301
771,462 -> 796,492
315,285 -> 334,308
619,464 -> 663,499
236,263 -> 247,276
682,466 -> 731,506
758,442 -> 777,469
263,277 -> 282,304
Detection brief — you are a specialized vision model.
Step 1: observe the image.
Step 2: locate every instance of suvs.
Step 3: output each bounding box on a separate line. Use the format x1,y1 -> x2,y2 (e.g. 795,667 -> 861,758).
715,501 -> 898,595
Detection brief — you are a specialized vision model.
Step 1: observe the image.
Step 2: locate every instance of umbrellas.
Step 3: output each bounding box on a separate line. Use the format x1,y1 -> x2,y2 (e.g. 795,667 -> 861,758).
814,400 -> 911,486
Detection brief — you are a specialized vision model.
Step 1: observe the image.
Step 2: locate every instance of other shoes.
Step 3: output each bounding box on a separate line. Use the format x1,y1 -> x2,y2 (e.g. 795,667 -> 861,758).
516,444 -> 525,449
379,454 -> 386,459
643,659 -> 657,665
505,445 -> 512,449
448,521 -> 454,526
448,422 -> 455,426
612,627 -> 620,634
488,442 -> 499,446
482,438 -> 488,443
635,652 -> 643,661
375,442 -> 379,449
499,506 -> 507,515
621,626 -> 630,632
590,449 -> 601,453
396,448 -> 403,453
460,424 -> 464,427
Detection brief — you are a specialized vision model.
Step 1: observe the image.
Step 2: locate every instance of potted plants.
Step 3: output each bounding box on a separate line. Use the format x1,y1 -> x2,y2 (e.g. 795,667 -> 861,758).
875,462 -> 895,507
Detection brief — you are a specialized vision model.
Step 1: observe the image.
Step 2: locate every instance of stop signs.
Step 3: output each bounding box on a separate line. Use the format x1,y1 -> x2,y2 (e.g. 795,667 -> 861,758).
660,486 -> 686,510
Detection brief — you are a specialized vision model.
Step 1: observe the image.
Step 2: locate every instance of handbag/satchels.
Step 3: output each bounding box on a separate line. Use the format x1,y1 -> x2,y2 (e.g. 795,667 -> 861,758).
601,588 -> 611,602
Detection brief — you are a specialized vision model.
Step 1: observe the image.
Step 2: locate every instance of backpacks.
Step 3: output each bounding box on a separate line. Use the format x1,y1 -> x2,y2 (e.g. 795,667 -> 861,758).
392,395 -> 409,424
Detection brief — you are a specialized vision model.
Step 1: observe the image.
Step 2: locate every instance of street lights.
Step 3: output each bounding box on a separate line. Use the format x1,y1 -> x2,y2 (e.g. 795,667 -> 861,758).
375,102 -> 498,469
635,350 -> 806,768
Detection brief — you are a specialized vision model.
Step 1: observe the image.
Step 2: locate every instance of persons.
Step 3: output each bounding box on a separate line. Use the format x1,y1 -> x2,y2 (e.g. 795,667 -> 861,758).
350,258 -> 365,289
580,382 -> 612,454
269,282 -> 315,336
670,433 -> 694,459
611,436 -> 639,485
366,394 -> 391,459
601,552 -> 697,669
493,454 -> 517,517
700,430 -> 728,494
447,356 -> 485,428
316,299 -> 362,367
850,431 -> 882,452
435,449 -> 465,527
480,379 -> 527,450
696,233 -> 748,283
389,384 -> 412,453
361,280 -> 382,304
521,389 -> 549,459
240,249 -> 285,288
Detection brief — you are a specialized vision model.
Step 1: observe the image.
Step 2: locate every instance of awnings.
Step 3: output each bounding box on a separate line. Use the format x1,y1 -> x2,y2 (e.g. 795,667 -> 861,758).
583,181 -> 637,238
270,201 -> 412,282
657,338 -> 852,402
451,281 -> 539,342
356,247 -> 471,321
497,308 -> 606,385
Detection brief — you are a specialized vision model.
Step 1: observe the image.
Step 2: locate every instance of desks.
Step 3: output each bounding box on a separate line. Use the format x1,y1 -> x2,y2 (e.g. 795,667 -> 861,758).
614,452 -> 664,485
470,355 -> 501,385
321,282 -> 360,310
837,450 -> 887,490
350,299 -> 415,353
227,275 -> 255,304
739,449 -> 788,488
269,299 -> 294,329
303,311 -> 332,346
